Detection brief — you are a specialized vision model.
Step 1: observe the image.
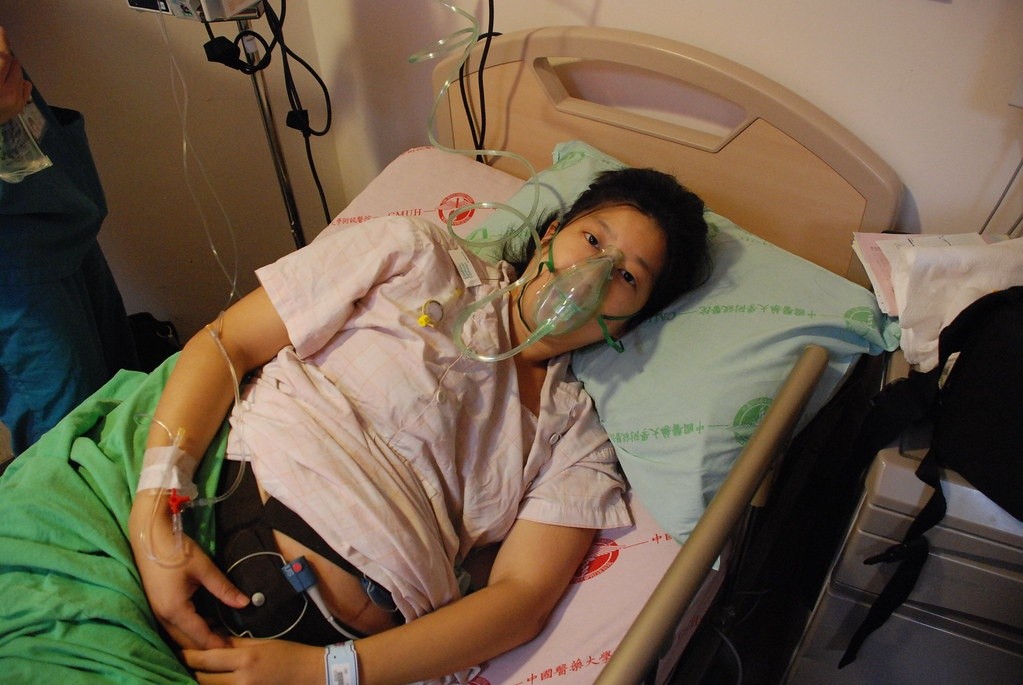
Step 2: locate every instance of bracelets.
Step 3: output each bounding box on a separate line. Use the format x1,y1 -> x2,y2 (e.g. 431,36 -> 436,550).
323,640 -> 359,685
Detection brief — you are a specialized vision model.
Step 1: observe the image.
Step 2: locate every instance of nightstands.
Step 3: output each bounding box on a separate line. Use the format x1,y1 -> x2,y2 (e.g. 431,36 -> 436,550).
780,431 -> 1023,685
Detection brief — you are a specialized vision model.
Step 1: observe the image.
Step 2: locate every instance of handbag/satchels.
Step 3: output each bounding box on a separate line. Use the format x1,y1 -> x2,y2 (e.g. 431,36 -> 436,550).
129,312 -> 182,372
837,286 -> 1023,669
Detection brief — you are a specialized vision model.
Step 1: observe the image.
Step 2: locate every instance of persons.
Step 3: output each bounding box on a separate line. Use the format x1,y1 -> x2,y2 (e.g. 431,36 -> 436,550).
0,169 -> 715,685
0,26 -> 141,459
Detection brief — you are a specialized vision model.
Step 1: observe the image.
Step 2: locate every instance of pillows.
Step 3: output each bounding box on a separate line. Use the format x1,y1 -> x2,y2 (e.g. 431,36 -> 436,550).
465,141 -> 900,570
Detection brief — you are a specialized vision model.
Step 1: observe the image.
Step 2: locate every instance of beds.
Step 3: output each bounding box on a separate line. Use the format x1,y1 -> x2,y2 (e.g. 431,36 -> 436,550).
0,0 -> 905,685
